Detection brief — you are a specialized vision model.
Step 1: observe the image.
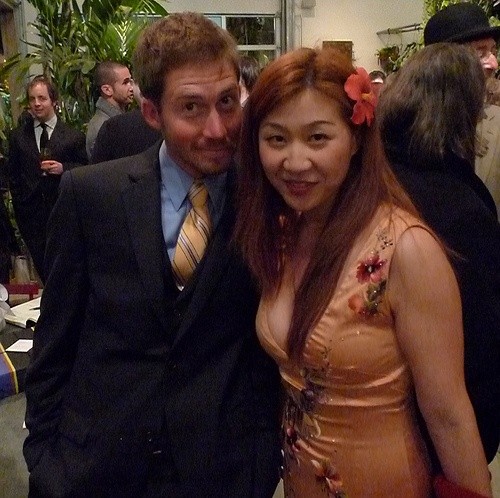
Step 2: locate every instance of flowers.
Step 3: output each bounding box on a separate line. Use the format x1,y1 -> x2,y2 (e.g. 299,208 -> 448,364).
345,67 -> 378,127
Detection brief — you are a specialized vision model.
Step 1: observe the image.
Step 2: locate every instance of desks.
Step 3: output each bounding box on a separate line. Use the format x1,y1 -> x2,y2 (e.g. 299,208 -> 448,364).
0,320 -> 35,497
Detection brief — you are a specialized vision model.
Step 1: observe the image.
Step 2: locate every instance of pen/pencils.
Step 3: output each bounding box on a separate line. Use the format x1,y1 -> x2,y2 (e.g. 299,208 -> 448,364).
30,307 -> 40,311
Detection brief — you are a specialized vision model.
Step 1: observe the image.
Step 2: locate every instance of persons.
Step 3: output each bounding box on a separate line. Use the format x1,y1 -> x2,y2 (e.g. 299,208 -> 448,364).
369,3 -> 500,498
238,56 -> 260,109
233,46 -> 492,498
23,13 -> 286,498
0,62 -> 162,306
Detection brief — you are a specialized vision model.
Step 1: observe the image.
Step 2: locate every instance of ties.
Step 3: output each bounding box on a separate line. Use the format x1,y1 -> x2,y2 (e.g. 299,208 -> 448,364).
38,123 -> 49,154
171,182 -> 211,286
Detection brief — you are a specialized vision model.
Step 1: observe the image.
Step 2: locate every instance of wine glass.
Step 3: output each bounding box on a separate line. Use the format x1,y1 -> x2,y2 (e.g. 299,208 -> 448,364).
39,147 -> 52,177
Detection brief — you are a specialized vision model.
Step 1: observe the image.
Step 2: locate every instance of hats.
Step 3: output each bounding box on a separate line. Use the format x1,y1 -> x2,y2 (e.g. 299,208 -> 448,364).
424,2 -> 500,48
